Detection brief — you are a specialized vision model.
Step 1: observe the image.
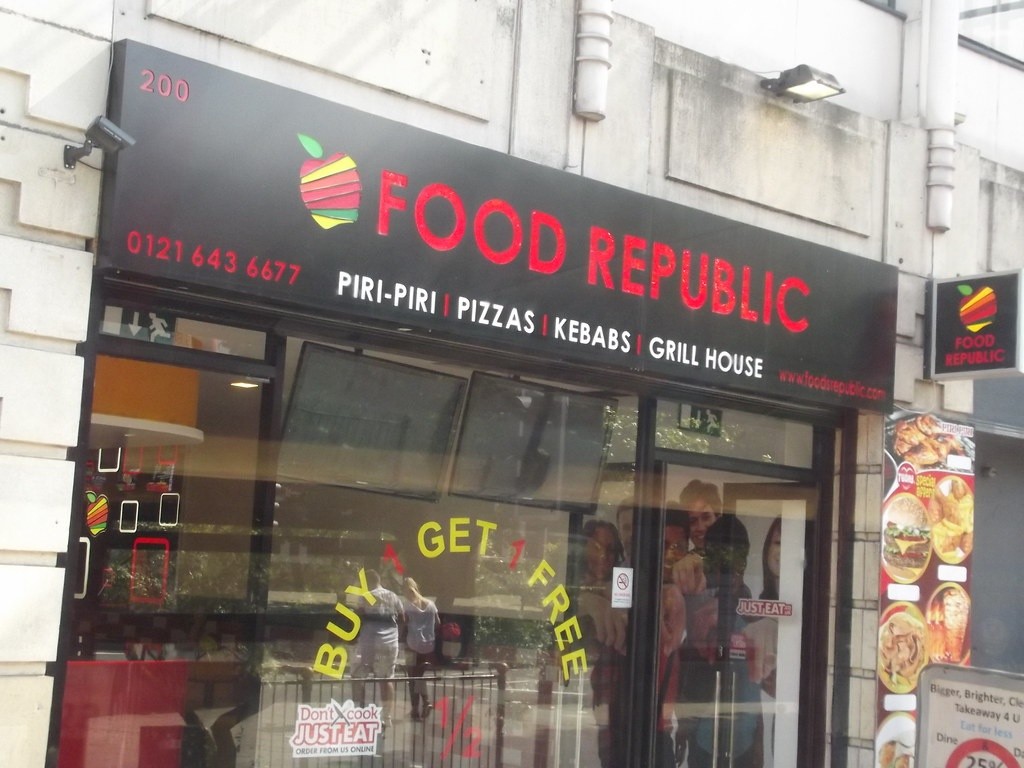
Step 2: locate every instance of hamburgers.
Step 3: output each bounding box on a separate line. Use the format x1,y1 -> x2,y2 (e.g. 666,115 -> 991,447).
883,497 -> 930,568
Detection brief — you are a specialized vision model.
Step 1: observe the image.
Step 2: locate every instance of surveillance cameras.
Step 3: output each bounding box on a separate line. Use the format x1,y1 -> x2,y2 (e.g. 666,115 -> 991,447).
84,116 -> 136,154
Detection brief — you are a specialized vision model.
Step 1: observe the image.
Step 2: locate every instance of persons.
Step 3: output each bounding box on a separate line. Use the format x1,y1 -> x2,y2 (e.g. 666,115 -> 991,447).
564,475 -> 806,768
345,569 -> 443,729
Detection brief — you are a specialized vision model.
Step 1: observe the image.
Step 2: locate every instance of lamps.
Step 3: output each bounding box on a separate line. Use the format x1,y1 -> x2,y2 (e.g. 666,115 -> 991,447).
64,115 -> 135,169
760,64 -> 846,104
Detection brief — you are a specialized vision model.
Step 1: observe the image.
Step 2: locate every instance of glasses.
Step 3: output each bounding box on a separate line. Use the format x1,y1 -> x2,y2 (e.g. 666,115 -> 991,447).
664,540 -> 688,556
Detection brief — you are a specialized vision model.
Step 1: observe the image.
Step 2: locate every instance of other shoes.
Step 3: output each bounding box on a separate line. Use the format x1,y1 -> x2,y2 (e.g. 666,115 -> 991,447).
381,715 -> 393,728
405,712 -> 421,721
421,700 -> 434,718
